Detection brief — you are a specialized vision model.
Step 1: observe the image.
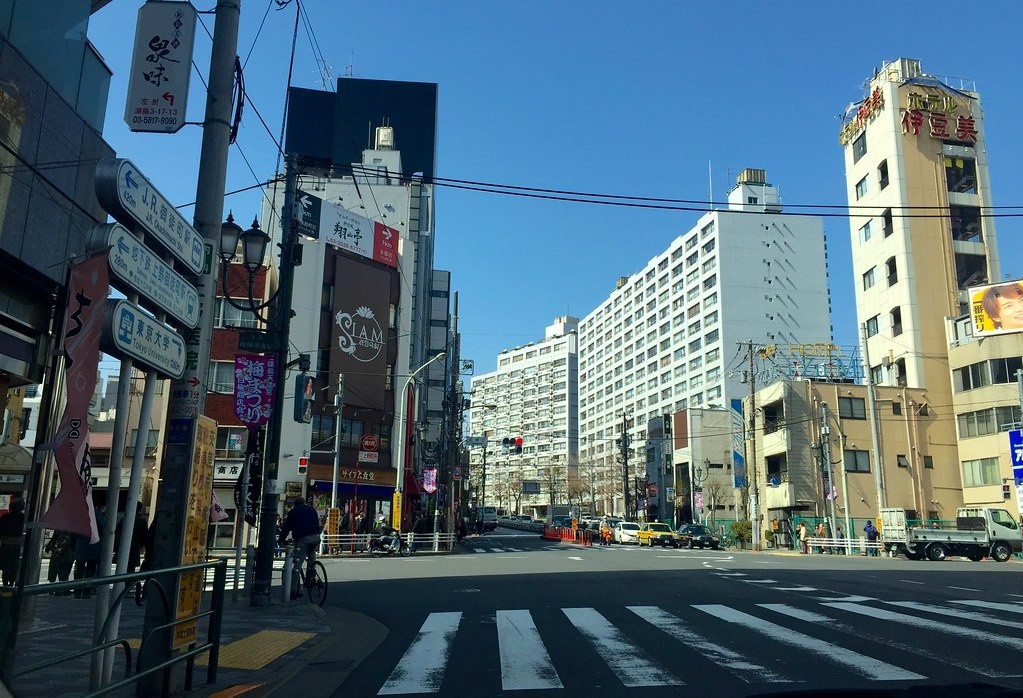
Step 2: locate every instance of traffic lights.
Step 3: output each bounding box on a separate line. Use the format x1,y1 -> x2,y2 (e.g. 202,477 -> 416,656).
516,446 -> 522,454
663,413 -> 671,435
502,437 -> 522,446
296,376 -> 317,423
1002,485 -> 1011,499
298,457 -> 308,476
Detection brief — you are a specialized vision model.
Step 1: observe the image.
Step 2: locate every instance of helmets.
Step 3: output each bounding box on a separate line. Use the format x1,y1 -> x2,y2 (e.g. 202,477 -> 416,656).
603,515 -> 607,518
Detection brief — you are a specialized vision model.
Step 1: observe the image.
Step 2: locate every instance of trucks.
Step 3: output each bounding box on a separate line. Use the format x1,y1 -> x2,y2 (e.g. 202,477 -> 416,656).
879,507 -> 941,556
906,506 -> 1023,562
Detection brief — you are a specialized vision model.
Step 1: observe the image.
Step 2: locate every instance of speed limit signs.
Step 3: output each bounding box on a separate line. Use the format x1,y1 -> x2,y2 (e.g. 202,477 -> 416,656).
453,466 -> 462,476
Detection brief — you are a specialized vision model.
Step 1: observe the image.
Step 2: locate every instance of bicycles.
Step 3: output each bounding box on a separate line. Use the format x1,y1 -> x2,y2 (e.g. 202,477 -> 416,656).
275,539 -> 328,607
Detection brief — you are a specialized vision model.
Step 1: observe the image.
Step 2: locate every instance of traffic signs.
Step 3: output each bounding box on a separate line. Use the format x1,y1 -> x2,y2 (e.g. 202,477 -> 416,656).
108,222 -> 201,329
113,300 -> 189,380
293,190 -> 400,270
117,157 -> 207,276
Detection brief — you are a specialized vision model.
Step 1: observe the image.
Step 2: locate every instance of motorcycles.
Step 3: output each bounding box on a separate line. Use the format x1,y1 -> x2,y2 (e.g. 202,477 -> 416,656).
367,529 -> 412,558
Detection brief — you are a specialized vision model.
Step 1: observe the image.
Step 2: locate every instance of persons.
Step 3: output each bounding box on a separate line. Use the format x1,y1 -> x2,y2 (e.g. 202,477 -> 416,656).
402,511 -> 434,550
599,516 -> 611,545
276,498 -> 321,598
982,283 -> 1023,330
796,523 -> 807,553
439,516 -> 468,549
0,499 -> 25,585
837,528 -> 845,554
45,501 -> 147,598
813,524 -> 826,553
863,520 -> 878,557
930,522 -> 940,529
477,520 -> 483,535
321,509 -> 392,553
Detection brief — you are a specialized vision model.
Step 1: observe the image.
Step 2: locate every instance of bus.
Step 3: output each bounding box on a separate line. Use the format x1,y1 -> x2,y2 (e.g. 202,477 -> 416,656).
464,506 -> 498,532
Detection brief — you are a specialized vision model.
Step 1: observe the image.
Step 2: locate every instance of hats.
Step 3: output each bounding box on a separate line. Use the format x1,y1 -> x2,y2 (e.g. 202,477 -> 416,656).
379,516 -> 385,520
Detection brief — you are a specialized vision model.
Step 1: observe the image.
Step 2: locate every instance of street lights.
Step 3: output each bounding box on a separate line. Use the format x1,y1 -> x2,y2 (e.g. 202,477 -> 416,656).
811,442 -> 831,538
692,456 -> 710,523
447,404 -> 497,552
707,402 -> 748,521
391,352 -> 448,531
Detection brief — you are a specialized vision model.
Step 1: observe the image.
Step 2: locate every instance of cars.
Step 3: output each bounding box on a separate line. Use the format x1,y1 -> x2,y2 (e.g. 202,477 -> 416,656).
636,522 -> 679,549
677,525 -> 719,550
498,513 -> 641,546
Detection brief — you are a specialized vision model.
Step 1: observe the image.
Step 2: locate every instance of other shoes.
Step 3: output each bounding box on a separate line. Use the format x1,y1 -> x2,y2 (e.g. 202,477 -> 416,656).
50,590 -> 134,599
290,591 -> 297,600
